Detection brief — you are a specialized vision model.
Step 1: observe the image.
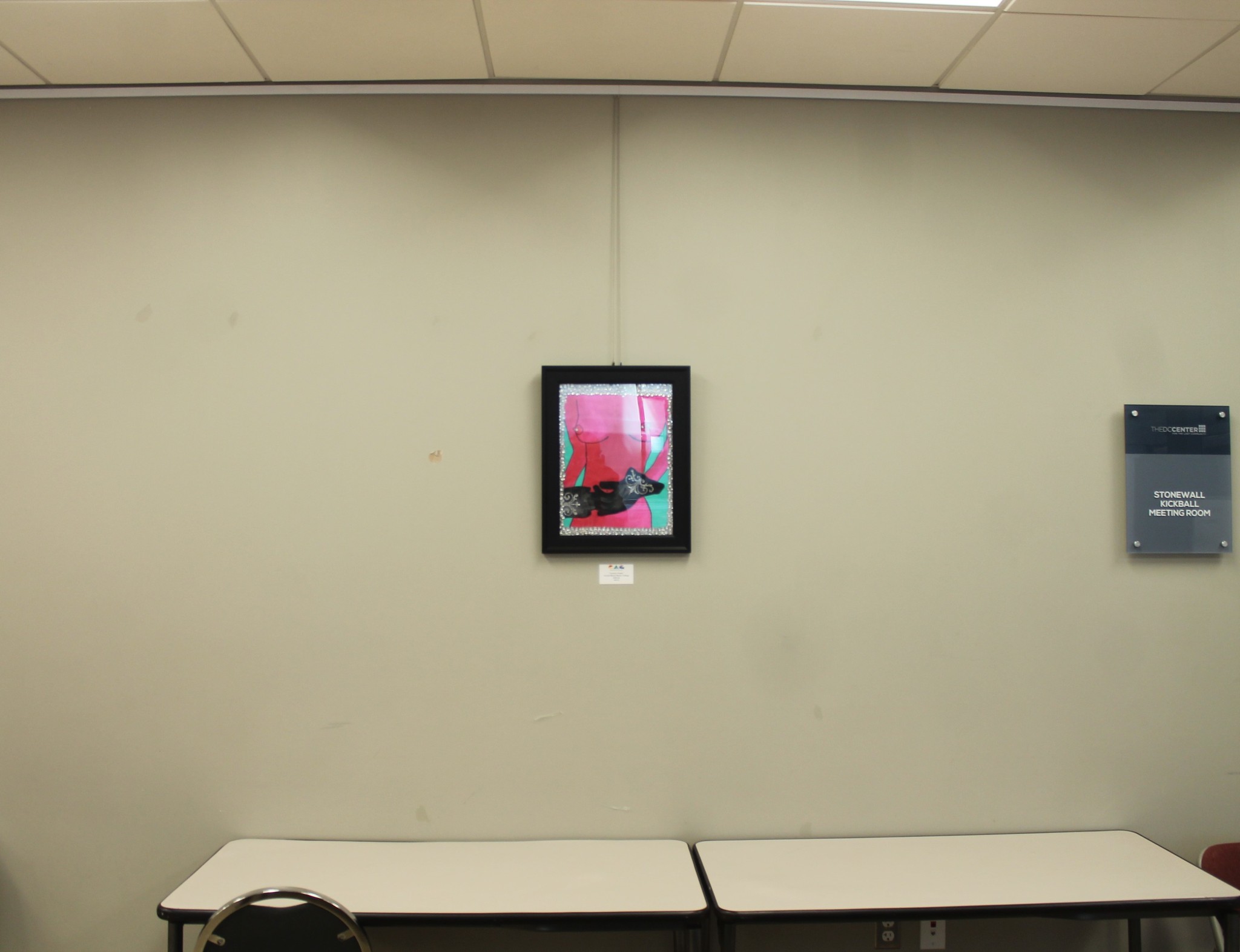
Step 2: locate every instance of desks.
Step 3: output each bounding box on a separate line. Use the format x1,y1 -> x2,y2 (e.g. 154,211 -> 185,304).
154,835 -> 711,952
692,825 -> 1240,952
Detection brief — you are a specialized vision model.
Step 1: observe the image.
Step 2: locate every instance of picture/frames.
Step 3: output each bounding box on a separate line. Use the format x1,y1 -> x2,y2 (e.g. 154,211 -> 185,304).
540,365 -> 693,554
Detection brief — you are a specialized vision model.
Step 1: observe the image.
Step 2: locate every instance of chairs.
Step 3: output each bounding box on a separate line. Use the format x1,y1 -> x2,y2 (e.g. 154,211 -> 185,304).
193,887 -> 372,952
